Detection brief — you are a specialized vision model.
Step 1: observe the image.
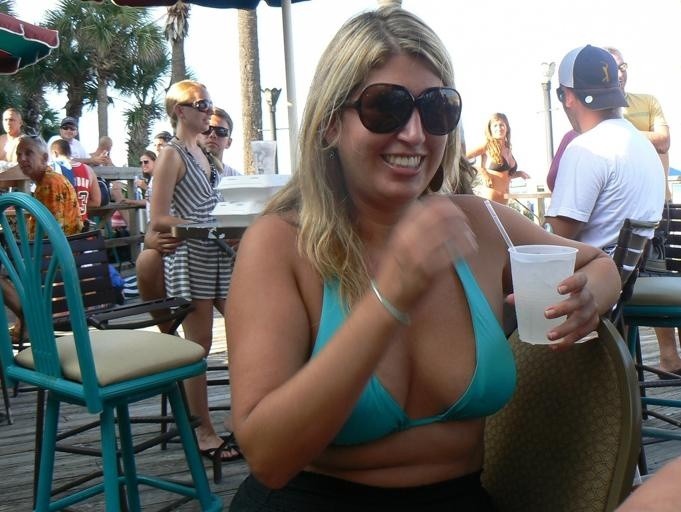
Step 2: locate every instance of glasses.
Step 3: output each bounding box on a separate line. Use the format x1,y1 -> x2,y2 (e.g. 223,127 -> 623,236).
201,126 -> 230,137
617,62 -> 629,71
140,160 -> 149,165
343,83 -> 462,135
62,125 -> 77,131
179,99 -> 212,112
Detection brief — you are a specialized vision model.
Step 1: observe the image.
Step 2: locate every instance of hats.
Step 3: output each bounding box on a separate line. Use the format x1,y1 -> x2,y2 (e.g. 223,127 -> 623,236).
558,45 -> 629,110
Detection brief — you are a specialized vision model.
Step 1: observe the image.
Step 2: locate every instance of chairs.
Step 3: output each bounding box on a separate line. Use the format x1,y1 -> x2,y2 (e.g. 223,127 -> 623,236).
619,276 -> 681,444
0,194 -> 222,512
10,228 -> 209,511
630,204 -> 681,430
612,216 -> 662,473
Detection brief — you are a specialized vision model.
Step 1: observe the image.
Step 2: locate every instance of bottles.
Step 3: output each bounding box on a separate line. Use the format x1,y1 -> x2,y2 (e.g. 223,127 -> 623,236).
134,176 -> 143,200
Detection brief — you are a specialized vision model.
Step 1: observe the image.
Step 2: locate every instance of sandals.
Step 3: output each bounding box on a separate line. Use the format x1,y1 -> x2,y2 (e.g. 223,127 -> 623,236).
198,442 -> 242,464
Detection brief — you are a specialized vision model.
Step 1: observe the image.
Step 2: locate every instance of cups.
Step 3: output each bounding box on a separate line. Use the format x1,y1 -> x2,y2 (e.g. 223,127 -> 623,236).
253,141 -> 277,175
508,244 -> 580,346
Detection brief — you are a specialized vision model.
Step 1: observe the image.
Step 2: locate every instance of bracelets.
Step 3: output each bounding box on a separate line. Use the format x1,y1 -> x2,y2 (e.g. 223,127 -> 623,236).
370,278 -> 412,327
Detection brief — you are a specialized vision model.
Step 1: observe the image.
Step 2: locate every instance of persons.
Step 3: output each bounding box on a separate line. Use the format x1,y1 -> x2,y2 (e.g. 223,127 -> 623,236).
137,106 -> 253,338
90,136 -> 117,167
584,48 -> 680,380
120,151 -> 159,225
47,117 -> 111,166
151,78 -> 240,462
51,140 -> 101,235
225,7 -> 619,512
0,135 -> 82,343
153,131 -> 172,155
545,44 -> 668,318
465,112 -> 532,204
0,107 -> 31,192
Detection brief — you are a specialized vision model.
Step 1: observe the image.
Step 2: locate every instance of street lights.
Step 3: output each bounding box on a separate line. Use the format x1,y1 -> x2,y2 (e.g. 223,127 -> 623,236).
537,59 -> 556,166
264,88 -> 280,175
